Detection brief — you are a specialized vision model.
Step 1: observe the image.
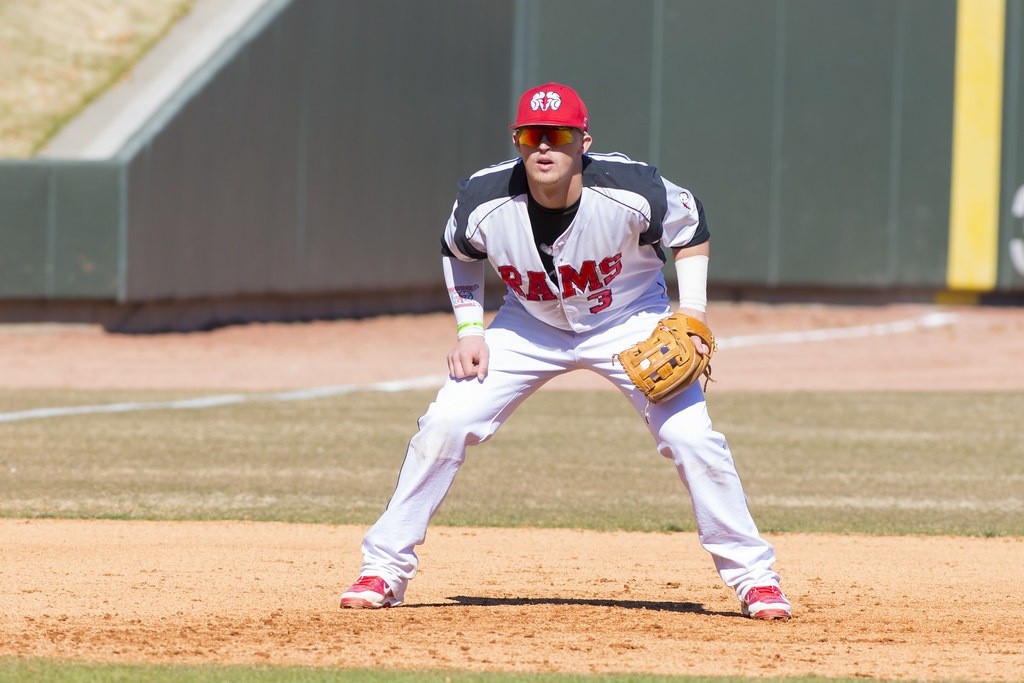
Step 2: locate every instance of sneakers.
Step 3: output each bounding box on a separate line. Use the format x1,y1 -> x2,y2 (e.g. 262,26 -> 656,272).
741,585 -> 791,620
340,576 -> 404,609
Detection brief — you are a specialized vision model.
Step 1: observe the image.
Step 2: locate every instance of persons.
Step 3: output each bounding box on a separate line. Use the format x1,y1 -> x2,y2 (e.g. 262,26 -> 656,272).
339,82 -> 791,619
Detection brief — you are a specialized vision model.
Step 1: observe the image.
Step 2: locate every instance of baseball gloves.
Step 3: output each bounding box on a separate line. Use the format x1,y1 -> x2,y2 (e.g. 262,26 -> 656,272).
611,312 -> 717,423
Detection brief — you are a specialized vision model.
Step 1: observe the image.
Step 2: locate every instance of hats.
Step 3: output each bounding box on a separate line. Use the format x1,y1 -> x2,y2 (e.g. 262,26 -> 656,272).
511,82 -> 589,132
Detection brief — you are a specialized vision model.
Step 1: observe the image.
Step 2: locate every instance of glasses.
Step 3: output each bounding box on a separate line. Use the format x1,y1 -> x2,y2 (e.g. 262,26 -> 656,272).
516,126 -> 584,148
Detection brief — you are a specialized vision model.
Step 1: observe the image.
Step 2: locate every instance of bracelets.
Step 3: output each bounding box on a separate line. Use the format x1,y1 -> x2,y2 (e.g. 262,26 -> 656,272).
457,322 -> 483,332
